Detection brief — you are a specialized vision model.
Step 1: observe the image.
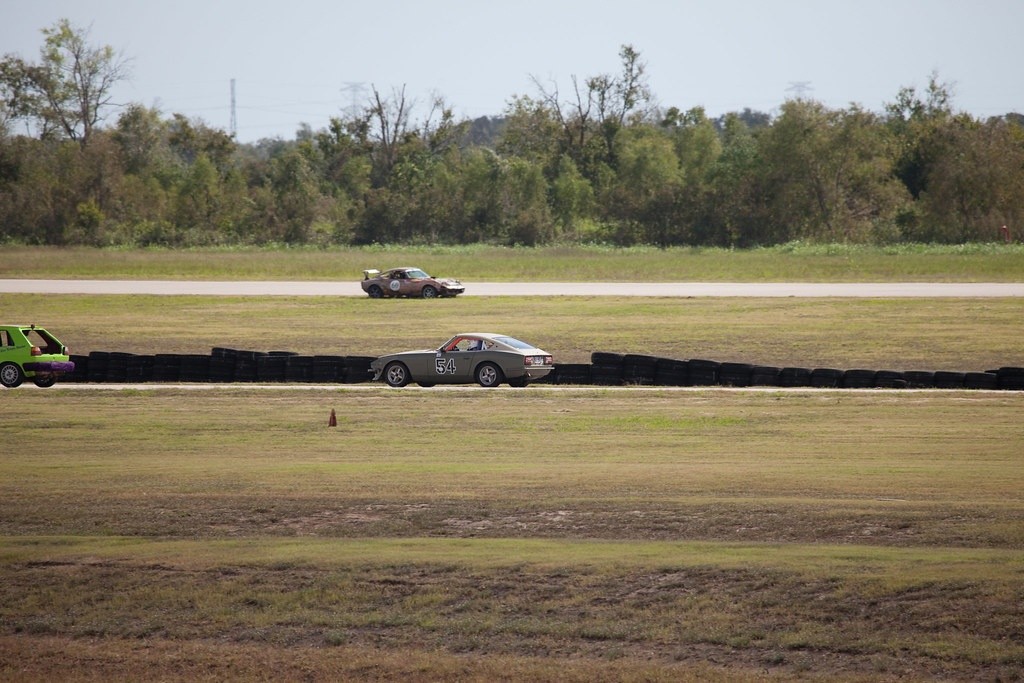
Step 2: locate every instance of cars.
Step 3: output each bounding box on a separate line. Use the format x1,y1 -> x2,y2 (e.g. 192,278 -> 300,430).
0,323 -> 75,389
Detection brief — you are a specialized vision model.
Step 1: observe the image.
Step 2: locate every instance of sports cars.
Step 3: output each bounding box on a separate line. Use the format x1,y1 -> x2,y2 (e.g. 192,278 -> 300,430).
361,265 -> 465,301
370,333 -> 554,388
